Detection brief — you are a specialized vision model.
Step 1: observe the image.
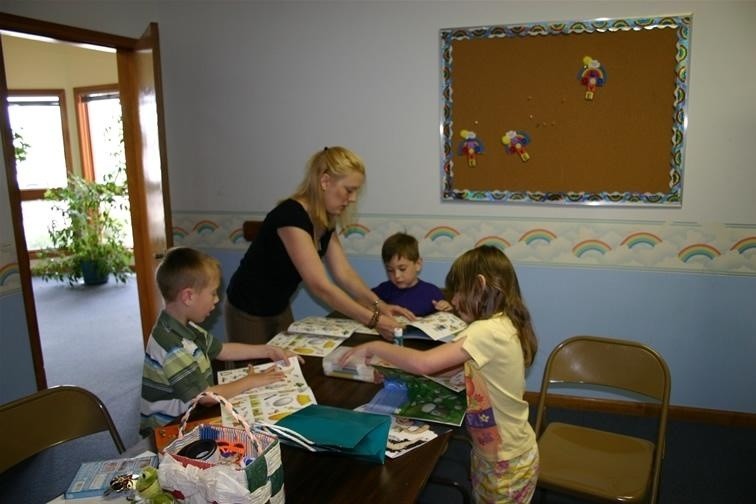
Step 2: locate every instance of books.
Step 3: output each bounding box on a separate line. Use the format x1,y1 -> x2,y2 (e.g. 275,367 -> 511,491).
216,311 -> 470,433
64,455 -> 158,500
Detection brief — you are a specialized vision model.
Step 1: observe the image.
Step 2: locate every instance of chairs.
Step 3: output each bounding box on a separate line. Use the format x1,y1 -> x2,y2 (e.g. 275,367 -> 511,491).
533,334 -> 672,504
0,382 -> 127,476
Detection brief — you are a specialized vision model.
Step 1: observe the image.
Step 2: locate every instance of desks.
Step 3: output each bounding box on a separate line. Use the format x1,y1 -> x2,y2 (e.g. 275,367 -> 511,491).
42,312 -> 455,504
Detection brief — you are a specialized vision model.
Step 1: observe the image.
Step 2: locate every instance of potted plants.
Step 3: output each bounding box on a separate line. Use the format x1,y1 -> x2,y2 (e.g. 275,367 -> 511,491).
30,164 -> 134,290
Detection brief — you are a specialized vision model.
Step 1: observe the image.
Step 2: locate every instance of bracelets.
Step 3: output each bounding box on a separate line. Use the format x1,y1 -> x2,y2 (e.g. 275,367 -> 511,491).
370,298 -> 383,310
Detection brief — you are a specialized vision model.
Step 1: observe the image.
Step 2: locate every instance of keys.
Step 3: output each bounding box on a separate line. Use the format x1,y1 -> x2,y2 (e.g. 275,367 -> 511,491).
102,474 -> 134,497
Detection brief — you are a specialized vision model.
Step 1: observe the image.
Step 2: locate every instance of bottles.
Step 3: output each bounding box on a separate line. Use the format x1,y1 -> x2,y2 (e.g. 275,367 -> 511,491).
392,326 -> 403,345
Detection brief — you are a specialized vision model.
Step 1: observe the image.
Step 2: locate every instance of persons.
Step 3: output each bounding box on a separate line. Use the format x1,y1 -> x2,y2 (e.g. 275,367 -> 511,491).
138,246 -> 305,440
370,232 -> 454,317
223,147 -> 417,368
335,245 -> 540,504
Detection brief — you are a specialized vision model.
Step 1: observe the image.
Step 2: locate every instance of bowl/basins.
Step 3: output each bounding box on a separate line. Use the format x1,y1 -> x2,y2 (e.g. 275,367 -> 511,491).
176,439 -> 220,465
